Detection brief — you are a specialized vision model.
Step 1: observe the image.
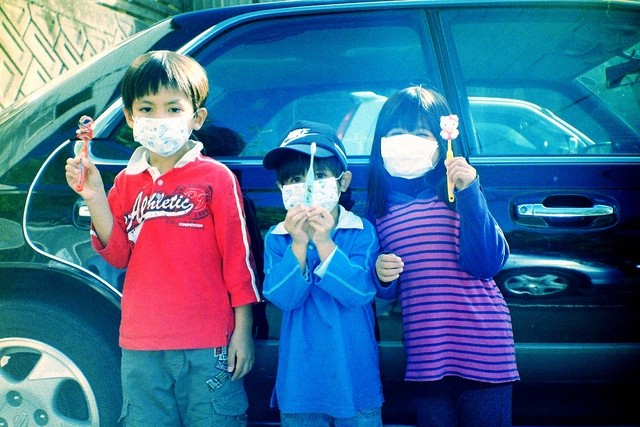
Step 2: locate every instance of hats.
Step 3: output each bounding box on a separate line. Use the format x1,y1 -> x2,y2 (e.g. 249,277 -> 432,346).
263,119 -> 348,172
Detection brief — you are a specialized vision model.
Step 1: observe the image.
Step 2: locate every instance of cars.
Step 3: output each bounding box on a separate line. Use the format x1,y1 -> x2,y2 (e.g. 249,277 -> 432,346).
1,1 -> 639,427
334,90 -> 597,165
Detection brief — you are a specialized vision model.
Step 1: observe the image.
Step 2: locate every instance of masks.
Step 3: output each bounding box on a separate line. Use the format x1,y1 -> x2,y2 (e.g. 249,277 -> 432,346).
282,171 -> 345,214
380,134 -> 443,180
128,106 -> 200,158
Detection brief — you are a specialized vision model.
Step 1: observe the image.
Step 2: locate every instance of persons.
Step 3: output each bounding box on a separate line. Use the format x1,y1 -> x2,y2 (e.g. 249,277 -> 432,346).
262,119 -> 385,426
66,48 -> 258,426
366,87 -> 520,427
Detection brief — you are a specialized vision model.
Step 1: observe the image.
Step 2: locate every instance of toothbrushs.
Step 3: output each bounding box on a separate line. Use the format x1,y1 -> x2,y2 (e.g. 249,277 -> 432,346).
304,141 -> 317,204
